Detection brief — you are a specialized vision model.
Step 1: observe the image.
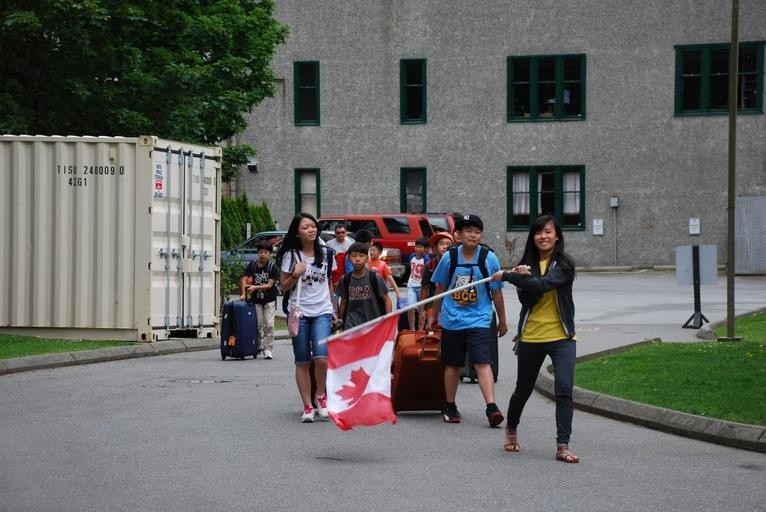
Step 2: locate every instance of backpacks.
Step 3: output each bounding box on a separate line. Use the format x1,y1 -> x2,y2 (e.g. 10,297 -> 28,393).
282,246 -> 336,313
368,260 -> 392,289
332,250 -> 349,294
444,241 -> 494,302
250,258 -> 281,296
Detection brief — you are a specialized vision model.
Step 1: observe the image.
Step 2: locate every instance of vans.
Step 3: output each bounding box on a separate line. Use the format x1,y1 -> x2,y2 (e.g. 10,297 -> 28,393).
426,211 -> 460,238
310,213 -> 436,273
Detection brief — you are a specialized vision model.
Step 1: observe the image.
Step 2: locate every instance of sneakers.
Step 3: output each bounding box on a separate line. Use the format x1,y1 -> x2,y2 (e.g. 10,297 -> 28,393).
441,404 -> 462,423
301,406 -> 314,422
263,349 -> 272,359
486,407 -> 504,426
313,394 -> 328,418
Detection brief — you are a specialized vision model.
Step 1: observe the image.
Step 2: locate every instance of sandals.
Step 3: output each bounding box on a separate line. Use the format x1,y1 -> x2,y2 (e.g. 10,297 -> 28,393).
504,424 -> 520,452
555,445 -> 579,463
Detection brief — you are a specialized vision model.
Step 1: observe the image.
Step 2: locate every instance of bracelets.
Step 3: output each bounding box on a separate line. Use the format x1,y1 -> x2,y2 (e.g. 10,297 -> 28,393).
291,273 -> 298,279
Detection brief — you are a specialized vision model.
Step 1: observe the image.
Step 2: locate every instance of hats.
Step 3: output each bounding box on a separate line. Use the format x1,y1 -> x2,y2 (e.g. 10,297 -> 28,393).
455,214 -> 483,232
429,232 -> 454,248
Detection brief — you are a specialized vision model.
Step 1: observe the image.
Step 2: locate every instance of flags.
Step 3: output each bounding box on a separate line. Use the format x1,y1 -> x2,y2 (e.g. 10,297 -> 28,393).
326,313 -> 400,431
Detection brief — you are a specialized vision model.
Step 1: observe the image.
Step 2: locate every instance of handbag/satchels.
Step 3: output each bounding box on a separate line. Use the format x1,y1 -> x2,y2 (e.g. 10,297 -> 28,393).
287,304 -> 304,338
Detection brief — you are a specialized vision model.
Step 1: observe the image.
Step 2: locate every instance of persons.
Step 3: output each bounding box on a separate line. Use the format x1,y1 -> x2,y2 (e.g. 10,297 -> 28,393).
491,215 -> 580,463
240,240 -> 277,359
425,214 -> 507,427
326,224 -> 461,331
280,212 -> 337,423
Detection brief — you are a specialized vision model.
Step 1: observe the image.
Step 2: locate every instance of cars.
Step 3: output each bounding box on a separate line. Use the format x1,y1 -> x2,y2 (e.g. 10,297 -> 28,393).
219,231 -> 406,287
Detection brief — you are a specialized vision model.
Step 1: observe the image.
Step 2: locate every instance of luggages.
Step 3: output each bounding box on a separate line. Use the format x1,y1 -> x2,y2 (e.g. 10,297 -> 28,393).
457,311 -> 499,384
220,285 -> 259,361
391,328 -> 450,414
396,298 -> 428,330
308,320 -> 344,409
